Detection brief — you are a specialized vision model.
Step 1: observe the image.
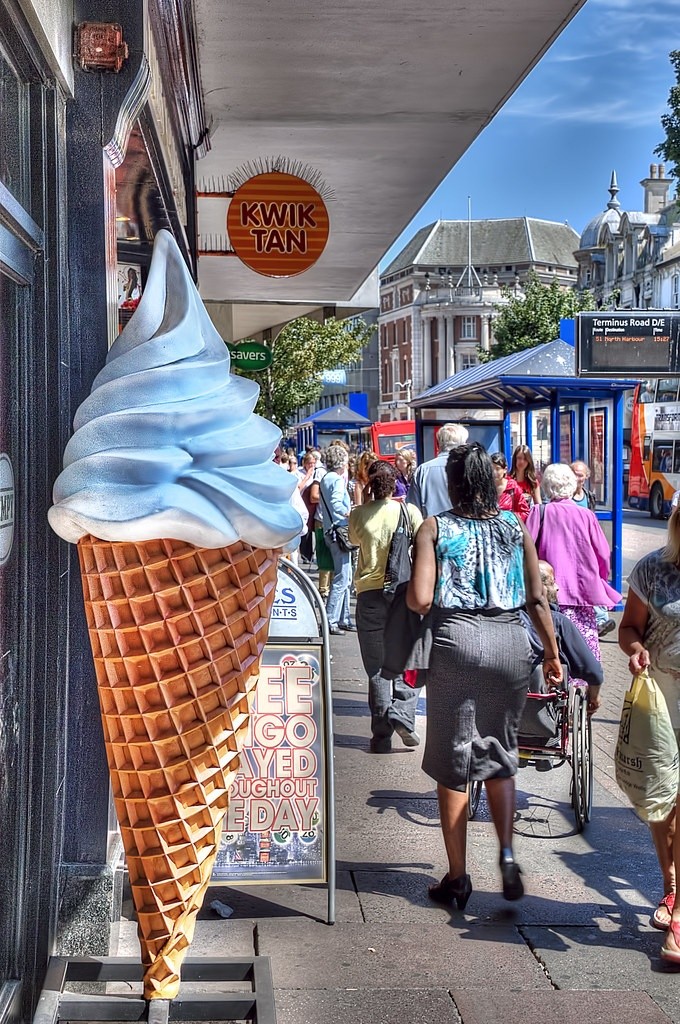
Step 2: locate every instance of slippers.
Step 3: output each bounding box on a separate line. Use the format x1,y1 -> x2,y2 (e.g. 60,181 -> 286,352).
660,920 -> 680,964
652,891 -> 678,931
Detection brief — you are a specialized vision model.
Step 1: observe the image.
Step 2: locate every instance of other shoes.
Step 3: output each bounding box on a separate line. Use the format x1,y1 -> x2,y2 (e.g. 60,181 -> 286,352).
395,722 -> 420,747
369,737 -> 391,752
534,747 -> 556,771
519,746 -> 534,768
597,619 -> 615,637
314,593 -> 329,608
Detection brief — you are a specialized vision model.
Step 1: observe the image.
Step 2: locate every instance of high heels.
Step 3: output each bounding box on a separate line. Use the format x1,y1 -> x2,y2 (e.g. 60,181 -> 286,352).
429,872 -> 472,911
502,863 -> 524,901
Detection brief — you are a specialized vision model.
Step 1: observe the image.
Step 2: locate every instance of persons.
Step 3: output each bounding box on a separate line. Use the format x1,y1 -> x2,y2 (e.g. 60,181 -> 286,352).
123,267 -> 141,301
516,560 -> 604,773
273,440 -> 418,635
618,489 -> 680,963
569,461 -> 615,637
524,462 -> 624,688
488,452 -> 529,524
405,443 -> 564,910
349,460 -> 425,755
404,424 -> 469,520
507,446 -> 542,505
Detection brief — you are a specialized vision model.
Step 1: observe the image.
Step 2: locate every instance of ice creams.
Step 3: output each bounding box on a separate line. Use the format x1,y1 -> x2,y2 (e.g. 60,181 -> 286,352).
45,224 -> 313,1005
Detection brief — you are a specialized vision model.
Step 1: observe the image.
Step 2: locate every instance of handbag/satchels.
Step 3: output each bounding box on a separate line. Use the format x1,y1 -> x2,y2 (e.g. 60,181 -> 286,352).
333,524 -> 360,551
382,500 -> 419,604
612,664 -> 680,822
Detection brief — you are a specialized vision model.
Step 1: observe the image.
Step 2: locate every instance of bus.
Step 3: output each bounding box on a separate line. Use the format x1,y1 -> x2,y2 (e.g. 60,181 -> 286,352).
621,378 -> 679,519
364,420 -> 442,469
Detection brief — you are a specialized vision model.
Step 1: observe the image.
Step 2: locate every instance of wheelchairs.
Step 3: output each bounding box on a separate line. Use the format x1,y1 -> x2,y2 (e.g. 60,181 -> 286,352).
466,664 -> 594,829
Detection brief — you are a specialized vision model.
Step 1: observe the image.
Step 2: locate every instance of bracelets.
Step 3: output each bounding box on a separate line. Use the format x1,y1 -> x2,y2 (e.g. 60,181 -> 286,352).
543,657 -> 560,660
